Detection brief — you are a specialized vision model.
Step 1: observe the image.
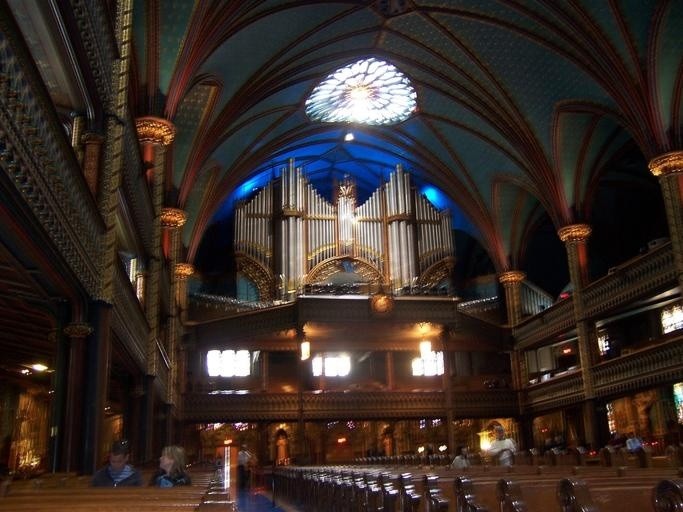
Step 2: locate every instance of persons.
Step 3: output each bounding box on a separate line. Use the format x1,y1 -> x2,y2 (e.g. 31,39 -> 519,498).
487,425 -> 517,467
450,444 -> 471,470
626,431 -> 641,454
146,444 -> 192,488
88,442 -> 143,487
236,443 -> 252,490
607,431 -> 623,449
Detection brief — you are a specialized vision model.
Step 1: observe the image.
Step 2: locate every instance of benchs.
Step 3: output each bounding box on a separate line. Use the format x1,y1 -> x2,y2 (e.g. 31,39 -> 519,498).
0,464 -> 238,512
